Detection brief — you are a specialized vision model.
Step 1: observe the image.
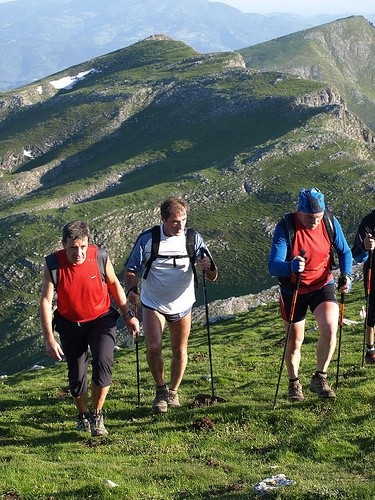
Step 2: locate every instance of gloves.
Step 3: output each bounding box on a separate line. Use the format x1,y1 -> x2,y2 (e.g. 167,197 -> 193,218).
290,256 -> 306,274
337,275 -> 354,294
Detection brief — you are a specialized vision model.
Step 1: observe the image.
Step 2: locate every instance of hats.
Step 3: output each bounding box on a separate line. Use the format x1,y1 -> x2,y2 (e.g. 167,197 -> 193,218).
298,186 -> 325,214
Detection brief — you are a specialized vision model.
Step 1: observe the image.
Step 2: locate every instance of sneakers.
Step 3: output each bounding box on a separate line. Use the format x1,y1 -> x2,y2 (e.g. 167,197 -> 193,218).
289,378 -> 304,402
168,388 -> 180,407
152,382 -> 169,414
308,371 -> 336,398
77,411 -> 91,432
365,352 -> 375,364
90,408 -> 108,436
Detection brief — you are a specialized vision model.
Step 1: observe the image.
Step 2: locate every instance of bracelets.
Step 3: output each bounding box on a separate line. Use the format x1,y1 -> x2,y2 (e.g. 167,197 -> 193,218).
125,286 -> 140,299
287,261 -> 292,273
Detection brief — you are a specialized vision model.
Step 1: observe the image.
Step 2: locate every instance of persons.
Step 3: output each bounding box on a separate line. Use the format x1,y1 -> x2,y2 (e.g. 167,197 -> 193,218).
122,197 -> 218,412
351,210 -> 375,363
268,186 -> 352,403
41,220 -> 140,436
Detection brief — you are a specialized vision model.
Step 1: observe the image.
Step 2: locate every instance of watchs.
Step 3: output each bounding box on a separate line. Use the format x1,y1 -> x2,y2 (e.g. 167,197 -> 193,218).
124,309 -> 135,321
